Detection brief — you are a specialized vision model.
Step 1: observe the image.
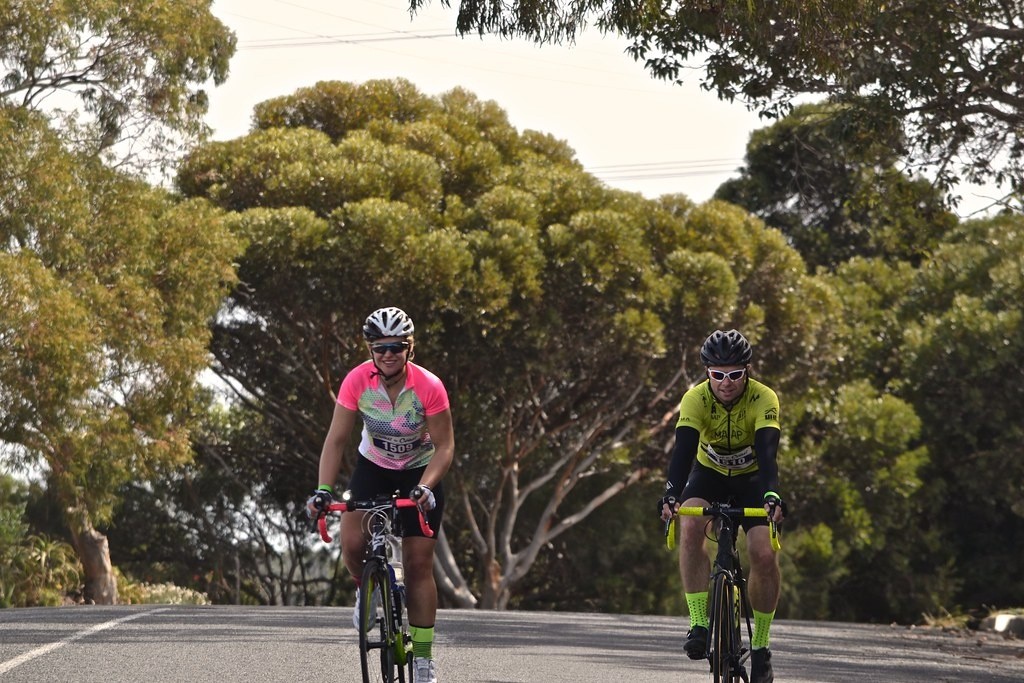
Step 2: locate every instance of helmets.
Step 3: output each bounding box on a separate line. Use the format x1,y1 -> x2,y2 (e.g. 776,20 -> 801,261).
363,307 -> 415,340
700,329 -> 753,366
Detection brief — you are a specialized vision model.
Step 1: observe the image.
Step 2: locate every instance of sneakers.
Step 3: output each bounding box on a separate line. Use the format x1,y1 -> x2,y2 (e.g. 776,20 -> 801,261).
413,657 -> 437,683
352,581 -> 380,632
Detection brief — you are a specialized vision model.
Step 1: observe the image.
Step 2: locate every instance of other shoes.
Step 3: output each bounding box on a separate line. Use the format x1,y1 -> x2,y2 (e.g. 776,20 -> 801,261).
683,623 -> 711,660
750,647 -> 774,683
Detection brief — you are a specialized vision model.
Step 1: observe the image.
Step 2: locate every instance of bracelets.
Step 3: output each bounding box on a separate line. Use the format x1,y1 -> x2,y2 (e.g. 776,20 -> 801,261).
764,491 -> 780,500
316,484 -> 334,495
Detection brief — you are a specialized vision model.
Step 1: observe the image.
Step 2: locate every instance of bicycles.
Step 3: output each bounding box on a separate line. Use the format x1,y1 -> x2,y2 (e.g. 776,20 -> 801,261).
311,490 -> 435,683
664,496 -> 782,683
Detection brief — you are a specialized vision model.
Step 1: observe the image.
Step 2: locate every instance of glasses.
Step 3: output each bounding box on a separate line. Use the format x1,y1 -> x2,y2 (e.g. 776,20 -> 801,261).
370,342 -> 409,353
708,368 -> 746,382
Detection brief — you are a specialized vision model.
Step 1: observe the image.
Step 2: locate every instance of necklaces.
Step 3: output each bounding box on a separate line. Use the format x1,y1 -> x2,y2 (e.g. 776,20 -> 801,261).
377,368 -> 407,390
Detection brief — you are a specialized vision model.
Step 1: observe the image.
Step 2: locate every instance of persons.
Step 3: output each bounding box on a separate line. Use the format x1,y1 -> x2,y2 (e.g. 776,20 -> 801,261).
305,306 -> 455,683
657,329 -> 788,683
